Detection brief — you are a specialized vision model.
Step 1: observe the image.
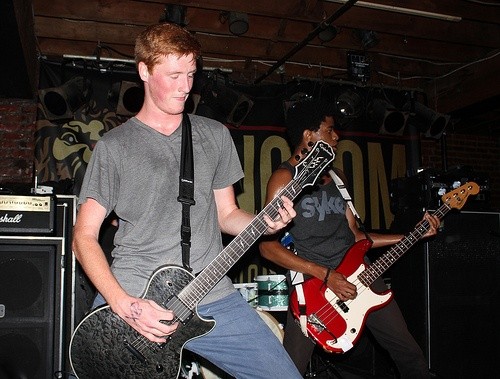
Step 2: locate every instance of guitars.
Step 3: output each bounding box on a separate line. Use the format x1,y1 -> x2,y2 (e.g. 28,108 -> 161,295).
290,180 -> 481,354
67,141 -> 336,379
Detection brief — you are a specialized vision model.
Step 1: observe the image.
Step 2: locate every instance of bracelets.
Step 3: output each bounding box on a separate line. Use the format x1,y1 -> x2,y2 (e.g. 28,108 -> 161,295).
324,267 -> 331,282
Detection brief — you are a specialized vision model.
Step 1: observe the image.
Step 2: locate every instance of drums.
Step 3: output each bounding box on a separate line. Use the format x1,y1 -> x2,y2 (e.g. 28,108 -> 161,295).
232,281 -> 258,309
256,275 -> 290,312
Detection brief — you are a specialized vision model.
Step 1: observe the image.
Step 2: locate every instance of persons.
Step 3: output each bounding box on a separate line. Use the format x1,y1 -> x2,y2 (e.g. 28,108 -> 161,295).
72,22 -> 305,379
259,99 -> 444,379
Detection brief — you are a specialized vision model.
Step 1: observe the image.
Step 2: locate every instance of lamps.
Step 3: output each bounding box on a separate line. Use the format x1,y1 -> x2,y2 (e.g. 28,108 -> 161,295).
184,93 -> 202,114
159,4 -> 189,28
36,59 -> 97,121
215,11 -> 249,35
282,74 -> 307,121
347,29 -> 380,83
330,84 -> 453,141
202,68 -> 256,126
103,63 -> 144,117
311,23 -> 342,45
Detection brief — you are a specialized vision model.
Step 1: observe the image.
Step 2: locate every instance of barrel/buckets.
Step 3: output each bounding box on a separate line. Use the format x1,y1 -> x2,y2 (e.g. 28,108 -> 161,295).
253,274 -> 289,311
232,283 -> 259,309
